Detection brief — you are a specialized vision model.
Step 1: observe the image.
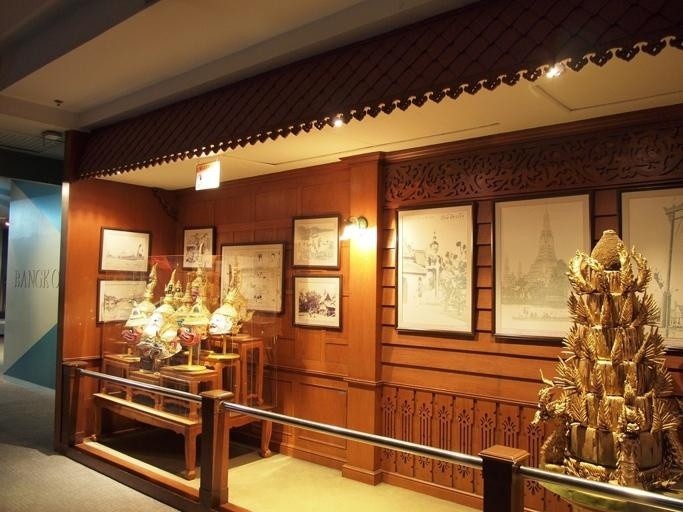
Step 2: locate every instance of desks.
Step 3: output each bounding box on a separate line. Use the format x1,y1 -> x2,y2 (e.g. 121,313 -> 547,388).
93,393 -> 277,480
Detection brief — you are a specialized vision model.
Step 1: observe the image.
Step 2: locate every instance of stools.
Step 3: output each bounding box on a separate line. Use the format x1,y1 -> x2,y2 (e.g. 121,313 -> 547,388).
102,335 -> 265,418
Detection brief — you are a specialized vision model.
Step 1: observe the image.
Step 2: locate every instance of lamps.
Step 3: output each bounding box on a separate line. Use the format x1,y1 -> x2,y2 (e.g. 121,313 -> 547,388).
340,216 -> 369,241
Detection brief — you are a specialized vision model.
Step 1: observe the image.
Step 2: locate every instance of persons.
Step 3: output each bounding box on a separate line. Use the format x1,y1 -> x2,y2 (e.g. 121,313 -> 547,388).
425,240 -> 442,274
136,243 -> 143,257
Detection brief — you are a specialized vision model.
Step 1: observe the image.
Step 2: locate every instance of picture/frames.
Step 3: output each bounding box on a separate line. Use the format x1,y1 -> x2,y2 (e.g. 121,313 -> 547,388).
182,227 -> 215,271
96,278 -> 152,325
290,213 -> 342,271
491,186 -> 595,344
395,201 -> 478,336
292,274 -> 343,330
219,244 -> 286,315
618,183 -> 683,354
98,226 -> 153,273
